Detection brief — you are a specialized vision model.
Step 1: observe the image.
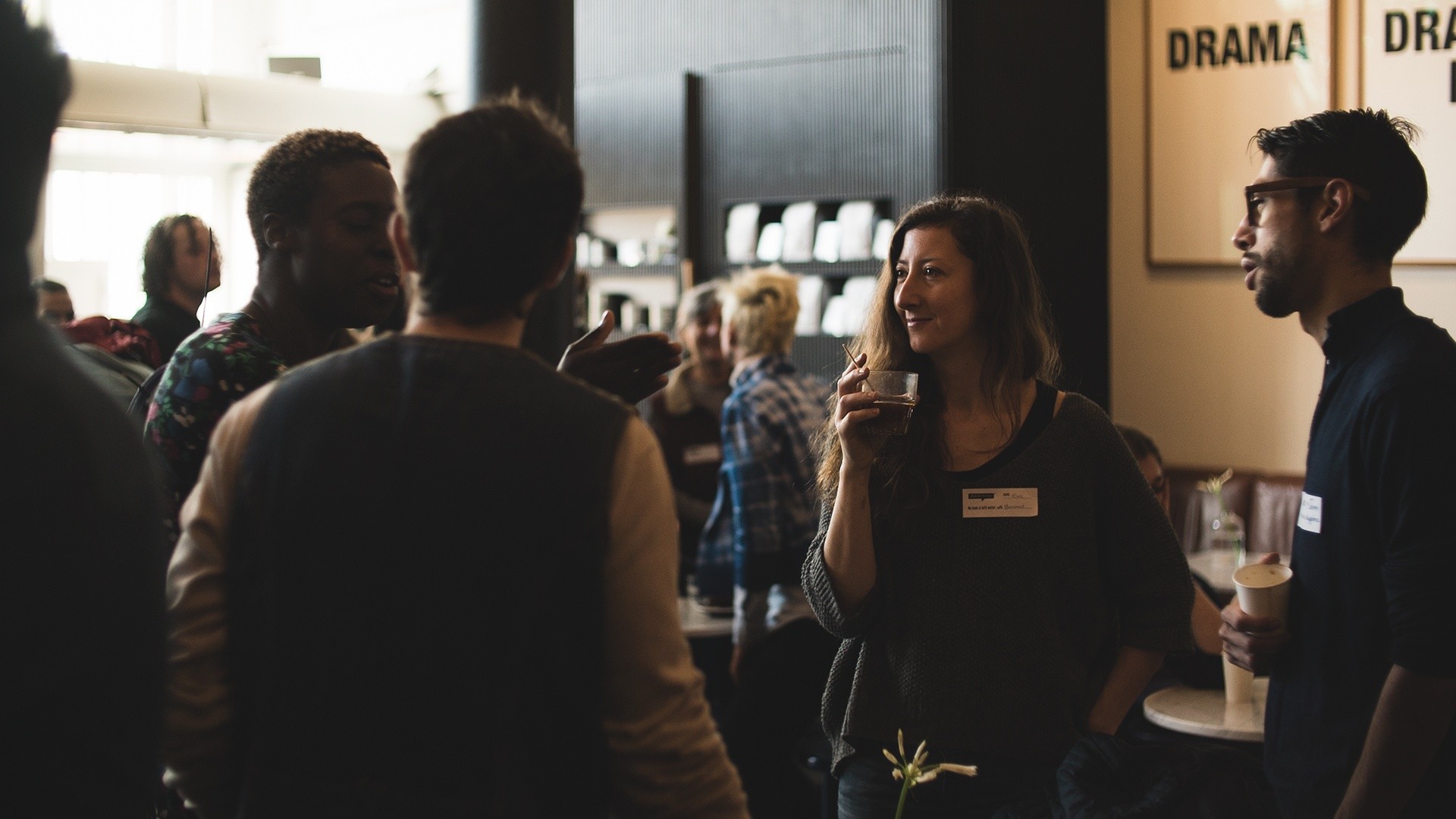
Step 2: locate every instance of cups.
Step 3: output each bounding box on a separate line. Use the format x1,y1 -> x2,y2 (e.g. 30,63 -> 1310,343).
857,371 -> 919,438
1232,563 -> 1293,639
1222,651 -> 1255,701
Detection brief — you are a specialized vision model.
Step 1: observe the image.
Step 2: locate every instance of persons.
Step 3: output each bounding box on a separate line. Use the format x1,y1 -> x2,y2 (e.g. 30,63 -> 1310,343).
154,94 -> 751,819
0,0 -> 687,819
637,265 -> 827,819
800,197 -> 1224,819
1217,109 -> 1456,819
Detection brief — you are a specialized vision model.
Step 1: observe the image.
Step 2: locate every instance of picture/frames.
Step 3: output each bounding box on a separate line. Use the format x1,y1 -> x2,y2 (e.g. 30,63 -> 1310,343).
1143,0 -> 1339,273
1358,1 -> 1456,266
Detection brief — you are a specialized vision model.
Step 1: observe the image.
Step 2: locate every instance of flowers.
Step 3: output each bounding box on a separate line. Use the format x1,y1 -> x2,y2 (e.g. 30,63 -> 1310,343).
883,729 -> 977,818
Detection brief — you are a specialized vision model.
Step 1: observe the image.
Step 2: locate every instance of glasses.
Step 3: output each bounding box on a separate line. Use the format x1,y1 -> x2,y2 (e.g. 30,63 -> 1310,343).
1244,174 -> 1373,227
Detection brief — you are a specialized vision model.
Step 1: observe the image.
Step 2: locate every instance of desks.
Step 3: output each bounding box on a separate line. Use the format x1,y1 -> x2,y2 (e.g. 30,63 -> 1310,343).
1184,548 -> 1292,590
1143,674 -> 1270,741
